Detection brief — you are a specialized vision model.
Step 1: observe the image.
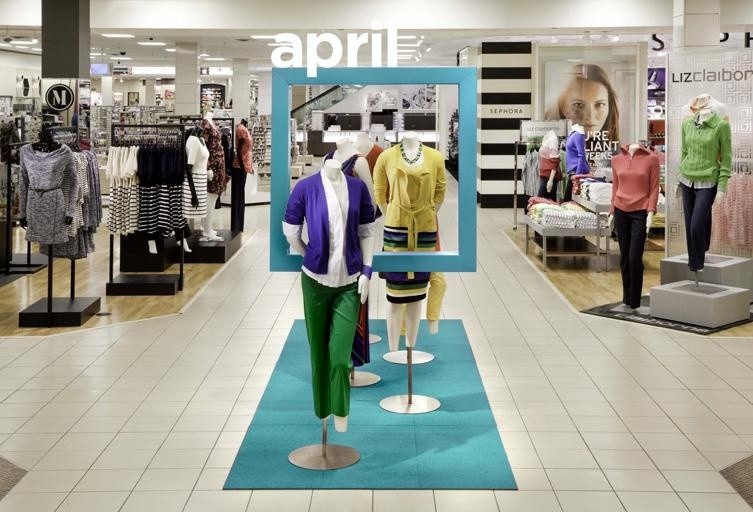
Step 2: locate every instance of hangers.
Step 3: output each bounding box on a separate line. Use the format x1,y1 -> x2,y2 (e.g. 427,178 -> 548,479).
52,133 -> 86,153
530,141 -> 566,153
109,129 -> 182,149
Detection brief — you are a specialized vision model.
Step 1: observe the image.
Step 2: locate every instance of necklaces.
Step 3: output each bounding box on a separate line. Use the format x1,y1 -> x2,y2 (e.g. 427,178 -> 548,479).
400,139 -> 422,164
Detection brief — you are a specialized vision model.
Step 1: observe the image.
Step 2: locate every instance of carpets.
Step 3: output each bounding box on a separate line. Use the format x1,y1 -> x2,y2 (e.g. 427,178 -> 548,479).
580,294 -> 753,336
223,318 -> 518,489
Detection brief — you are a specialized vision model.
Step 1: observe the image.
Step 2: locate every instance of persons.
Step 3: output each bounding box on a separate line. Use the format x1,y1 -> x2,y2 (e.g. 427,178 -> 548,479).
399,213 -> 447,336
321,137 -> 374,206
146,226 -> 192,254
607,144 -> 660,311
182,125 -> 210,218
545,64 -> 620,149
350,132 -> 386,252
674,109 -> 732,274
681,93 -> 729,124
282,160 -> 375,432
230,118 -> 255,233
199,112 -> 226,242
563,123 -> 591,203
18,129 -> 79,246
537,129 -> 562,202
373,132 -> 447,352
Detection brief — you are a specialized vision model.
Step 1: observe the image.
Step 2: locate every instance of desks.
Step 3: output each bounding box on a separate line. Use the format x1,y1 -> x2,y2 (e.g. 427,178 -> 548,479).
524,214 -> 611,272
